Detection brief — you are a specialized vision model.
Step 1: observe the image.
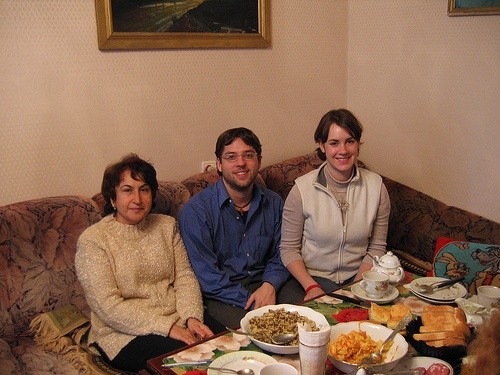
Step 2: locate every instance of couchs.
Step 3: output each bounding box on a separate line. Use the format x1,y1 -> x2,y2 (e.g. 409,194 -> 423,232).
0,152 -> 500,375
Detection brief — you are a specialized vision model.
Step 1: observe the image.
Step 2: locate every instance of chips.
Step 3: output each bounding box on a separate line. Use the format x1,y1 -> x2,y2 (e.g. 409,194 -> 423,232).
326,329 -> 394,366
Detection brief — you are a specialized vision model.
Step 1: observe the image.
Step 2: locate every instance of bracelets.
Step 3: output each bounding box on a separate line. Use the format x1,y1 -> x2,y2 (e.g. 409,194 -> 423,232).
306,285 -> 322,295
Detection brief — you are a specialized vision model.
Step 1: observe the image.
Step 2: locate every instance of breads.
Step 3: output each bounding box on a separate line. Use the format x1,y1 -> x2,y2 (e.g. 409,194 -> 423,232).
369,302 -> 412,336
412,305 -> 471,346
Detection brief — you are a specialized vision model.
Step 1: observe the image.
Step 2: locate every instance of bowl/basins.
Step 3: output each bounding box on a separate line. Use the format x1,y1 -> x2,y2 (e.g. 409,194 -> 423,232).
327,321 -> 408,374
241,303 -> 329,354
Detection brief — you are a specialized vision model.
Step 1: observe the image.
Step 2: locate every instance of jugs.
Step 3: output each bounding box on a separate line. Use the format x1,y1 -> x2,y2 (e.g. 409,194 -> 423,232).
454,298 -> 499,337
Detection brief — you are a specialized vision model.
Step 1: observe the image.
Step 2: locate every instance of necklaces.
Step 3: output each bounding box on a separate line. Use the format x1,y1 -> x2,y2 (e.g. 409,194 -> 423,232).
323,173 -> 349,214
233,195 -> 254,216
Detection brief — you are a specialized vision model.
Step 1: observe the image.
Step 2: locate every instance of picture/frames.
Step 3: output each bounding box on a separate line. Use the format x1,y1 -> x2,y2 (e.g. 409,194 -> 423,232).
447,0 -> 500,16
95,0 -> 270,49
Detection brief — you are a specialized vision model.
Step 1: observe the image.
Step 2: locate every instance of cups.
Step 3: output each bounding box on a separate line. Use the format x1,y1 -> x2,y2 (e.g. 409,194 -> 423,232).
259,362 -> 300,375
298,338 -> 330,375
362,270 -> 390,299
477,286 -> 500,312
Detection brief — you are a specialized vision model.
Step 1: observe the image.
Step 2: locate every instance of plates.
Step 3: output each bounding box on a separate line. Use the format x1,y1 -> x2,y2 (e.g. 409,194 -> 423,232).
392,356 -> 453,375
207,350 -> 278,375
410,277 -> 468,304
350,281 -> 399,304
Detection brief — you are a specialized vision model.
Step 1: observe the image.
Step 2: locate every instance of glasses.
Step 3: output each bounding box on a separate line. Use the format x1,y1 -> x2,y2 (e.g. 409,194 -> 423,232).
219,152 -> 257,160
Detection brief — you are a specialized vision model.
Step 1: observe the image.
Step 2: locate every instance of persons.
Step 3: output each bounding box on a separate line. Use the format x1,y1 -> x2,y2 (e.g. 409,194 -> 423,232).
179,128 -> 305,332
75,153 -> 226,375
283,110 -> 392,303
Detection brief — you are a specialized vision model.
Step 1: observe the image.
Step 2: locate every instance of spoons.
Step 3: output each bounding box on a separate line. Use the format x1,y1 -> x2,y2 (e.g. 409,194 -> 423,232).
362,320 -> 408,364
226,325 -> 296,344
205,362 -> 254,375
416,277 -> 465,295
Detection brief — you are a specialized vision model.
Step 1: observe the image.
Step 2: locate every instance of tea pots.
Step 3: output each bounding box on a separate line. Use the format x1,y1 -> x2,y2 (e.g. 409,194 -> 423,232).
370,252 -> 406,283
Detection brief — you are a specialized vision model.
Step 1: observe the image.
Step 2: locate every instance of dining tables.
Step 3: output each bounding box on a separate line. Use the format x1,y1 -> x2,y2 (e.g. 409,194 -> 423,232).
146,269 -> 464,374
